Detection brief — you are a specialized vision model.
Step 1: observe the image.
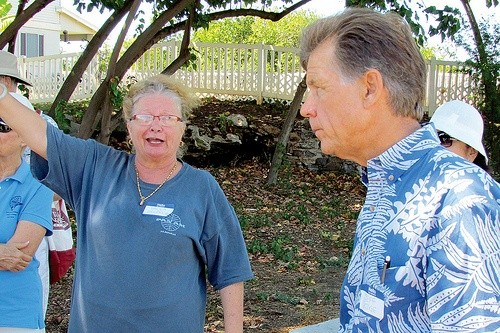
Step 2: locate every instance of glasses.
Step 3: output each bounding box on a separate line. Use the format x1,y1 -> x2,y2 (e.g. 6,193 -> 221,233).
437,131 -> 458,147
130,113 -> 183,127
0,118 -> 12,132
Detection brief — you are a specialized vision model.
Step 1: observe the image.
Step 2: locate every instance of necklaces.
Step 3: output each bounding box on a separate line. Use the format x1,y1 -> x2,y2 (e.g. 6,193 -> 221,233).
134,158 -> 177,206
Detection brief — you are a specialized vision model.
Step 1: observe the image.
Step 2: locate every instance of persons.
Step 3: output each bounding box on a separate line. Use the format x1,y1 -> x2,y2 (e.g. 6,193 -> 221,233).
0,51 -> 63,333
429,98 -> 487,172
298,9 -> 500,333
0,75 -> 254,333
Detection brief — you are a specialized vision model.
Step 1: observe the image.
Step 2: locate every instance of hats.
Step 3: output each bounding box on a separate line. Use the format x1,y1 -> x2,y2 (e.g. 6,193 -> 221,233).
0,51 -> 33,87
430,100 -> 488,166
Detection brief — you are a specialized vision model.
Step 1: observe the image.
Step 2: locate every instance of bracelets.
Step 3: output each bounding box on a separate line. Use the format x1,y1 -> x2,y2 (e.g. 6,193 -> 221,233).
0,83 -> 7,99
